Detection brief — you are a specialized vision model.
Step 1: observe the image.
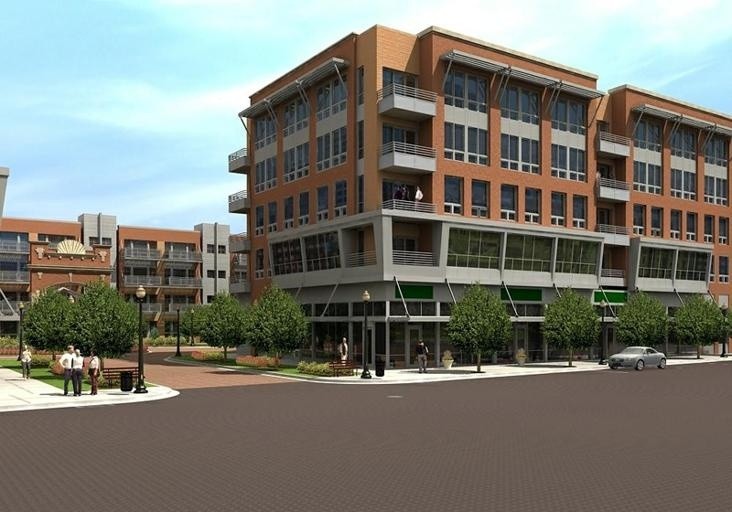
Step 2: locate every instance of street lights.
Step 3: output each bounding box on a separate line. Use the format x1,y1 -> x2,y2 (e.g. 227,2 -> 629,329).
599,298 -> 607,365
17,301 -> 24,361
134,283 -> 149,392
191,308 -> 195,345
175,304 -> 182,356
361,289 -> 372,378
68,294 -> 75,304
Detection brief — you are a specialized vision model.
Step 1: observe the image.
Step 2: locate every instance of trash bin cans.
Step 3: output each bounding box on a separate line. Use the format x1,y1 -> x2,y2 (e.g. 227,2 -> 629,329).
120,370 -> 133,391
375,360 -> 385,376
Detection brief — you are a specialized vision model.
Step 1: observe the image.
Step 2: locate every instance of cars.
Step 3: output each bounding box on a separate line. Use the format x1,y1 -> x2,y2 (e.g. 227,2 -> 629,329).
609,346 -> 667,371
